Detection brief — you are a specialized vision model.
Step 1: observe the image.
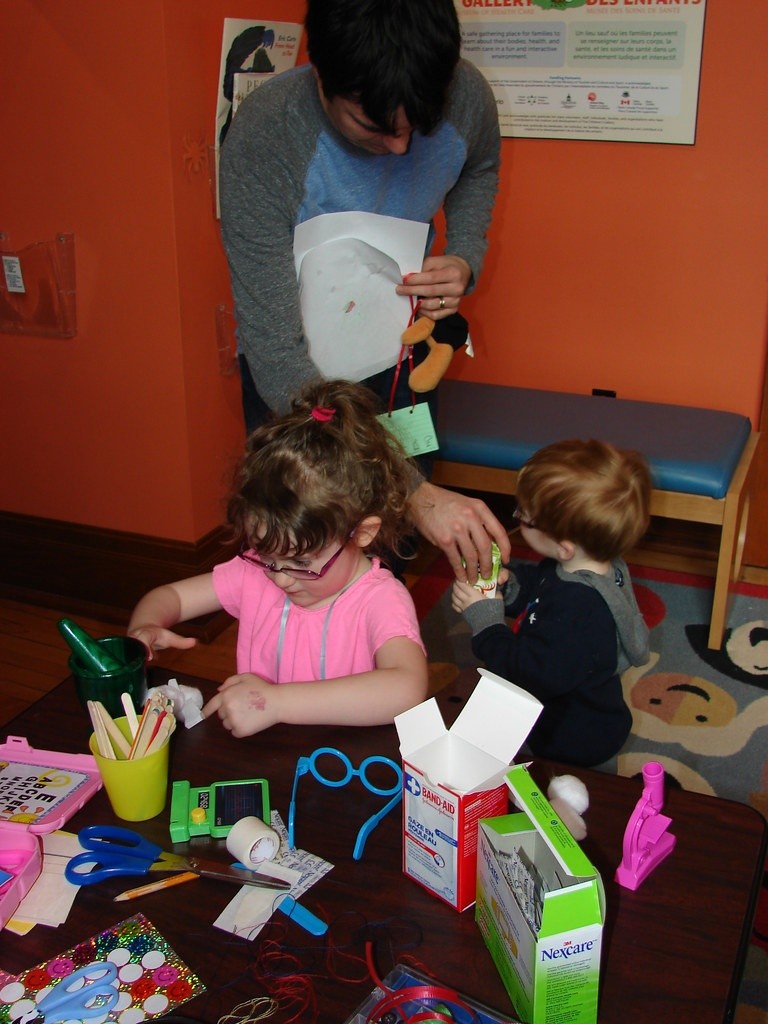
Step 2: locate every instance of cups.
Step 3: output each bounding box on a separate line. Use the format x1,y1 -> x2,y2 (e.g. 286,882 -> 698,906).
69,634 -> 147,729
89,715 -> 171,822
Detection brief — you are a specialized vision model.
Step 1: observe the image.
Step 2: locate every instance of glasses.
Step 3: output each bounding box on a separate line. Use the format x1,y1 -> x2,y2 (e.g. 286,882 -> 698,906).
237,517 -> 367,581
511,504 -> 577,544
288,747 -> 403,859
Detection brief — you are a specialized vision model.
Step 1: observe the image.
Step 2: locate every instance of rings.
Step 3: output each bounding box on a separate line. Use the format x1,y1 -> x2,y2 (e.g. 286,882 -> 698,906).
439,297 -> 446,308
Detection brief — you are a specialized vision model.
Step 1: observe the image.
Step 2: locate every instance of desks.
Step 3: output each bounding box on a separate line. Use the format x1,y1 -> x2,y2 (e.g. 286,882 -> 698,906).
0,659 -> 768,1024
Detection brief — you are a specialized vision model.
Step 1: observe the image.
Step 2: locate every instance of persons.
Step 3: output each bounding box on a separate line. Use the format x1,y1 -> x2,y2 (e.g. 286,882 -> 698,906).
126,379 -> 428,737
451,436 -> 651,766
216,0 -> 512,581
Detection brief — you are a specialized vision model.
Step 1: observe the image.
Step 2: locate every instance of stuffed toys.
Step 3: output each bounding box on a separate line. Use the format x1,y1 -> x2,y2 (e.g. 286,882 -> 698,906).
403,311 -> 473,393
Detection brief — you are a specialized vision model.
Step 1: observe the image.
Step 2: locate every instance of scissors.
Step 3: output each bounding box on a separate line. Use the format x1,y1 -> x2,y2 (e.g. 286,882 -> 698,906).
62,819 -> 292,894
11,960 -> 122,1023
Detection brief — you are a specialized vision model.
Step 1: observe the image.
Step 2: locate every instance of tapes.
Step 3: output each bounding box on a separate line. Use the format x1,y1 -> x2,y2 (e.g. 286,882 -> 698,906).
224,815 -> 282,871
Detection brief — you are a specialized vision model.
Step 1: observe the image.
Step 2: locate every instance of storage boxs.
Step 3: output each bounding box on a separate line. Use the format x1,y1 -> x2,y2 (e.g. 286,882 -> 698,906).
475,767 -> 607,1024
397,666 -> 544,913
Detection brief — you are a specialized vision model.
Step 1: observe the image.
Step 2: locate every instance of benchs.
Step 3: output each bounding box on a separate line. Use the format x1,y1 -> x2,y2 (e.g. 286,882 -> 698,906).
417,378 -> 760,650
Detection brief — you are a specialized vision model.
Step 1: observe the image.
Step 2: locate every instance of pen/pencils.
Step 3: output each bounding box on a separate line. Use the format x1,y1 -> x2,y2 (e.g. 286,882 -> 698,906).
111,870 -> 200,903
